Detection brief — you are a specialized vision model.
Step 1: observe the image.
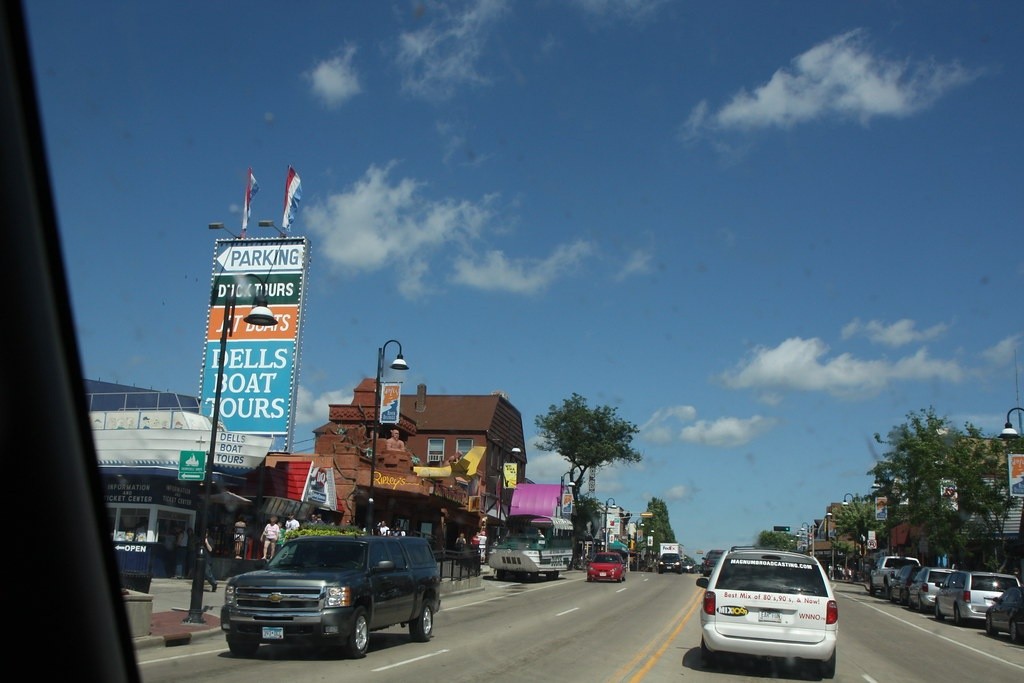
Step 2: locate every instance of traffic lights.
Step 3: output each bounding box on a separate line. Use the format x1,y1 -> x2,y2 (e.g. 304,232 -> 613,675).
774,525 -> 791,532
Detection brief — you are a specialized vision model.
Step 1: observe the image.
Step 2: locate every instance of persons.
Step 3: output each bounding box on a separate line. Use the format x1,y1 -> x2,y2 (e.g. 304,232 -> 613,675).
471,531 -> 487,565
309,514 -> 325,525
374,520 -> 405,537
444,451 -> 463,467
173,525 -> 188,580
828,566 -> 854,581
284,513 -> 301,543
344,520 -> 352,527
186,527 -> 200,580
260,516 -> 281,559
233,515 -> 246,560
538,529 -> 547,545
275,521 -> 286,553
204,527 -> 218,593
456,533 -> 467,567
386,429 -> 405,452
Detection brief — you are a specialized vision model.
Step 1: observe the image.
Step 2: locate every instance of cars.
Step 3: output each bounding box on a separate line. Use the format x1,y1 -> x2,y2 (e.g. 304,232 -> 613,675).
889,565 -> 927,606
658,553 -> 683,574
693,545 -> 840,681
984,586 -> 1024,641
586,551 -> 627,584
682,561 -> 702,574
908,566 -> 959,613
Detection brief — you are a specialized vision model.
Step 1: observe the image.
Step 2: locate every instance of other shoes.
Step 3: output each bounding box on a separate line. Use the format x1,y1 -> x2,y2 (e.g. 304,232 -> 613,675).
261,557 -> 266,559
211,584 -> 217,592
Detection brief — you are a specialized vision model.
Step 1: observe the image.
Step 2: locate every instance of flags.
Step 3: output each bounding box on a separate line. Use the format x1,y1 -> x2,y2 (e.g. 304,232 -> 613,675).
281,164 -> 303,233
240,167 -> 260,231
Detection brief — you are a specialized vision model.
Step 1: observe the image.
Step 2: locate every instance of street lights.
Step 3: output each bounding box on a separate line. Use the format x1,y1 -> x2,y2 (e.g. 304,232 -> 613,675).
603,497 -> 618,551
496,447 -> 521,542
365,338 -> 410,538
175,271 -> 284,626
558,471 -> 577,521
842,493 -> 865,582
799,522 -> 809,555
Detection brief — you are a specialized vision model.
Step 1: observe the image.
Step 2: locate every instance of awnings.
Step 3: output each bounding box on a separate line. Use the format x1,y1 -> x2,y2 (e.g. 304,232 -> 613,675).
607,540 -> 629,553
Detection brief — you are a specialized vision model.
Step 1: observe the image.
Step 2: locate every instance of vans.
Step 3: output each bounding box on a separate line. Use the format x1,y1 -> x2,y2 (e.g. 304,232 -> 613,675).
487,516 -> 577,583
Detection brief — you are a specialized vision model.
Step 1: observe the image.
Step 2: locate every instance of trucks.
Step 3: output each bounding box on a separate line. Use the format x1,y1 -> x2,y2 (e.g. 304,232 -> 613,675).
658,543 -> 682,557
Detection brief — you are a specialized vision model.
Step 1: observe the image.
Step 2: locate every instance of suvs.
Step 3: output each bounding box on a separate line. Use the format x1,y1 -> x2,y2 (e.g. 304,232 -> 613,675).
932,569 -> 1021,628
700,549 -> 725,577
220,532 -> 444,660
869,555 -> 922,600
